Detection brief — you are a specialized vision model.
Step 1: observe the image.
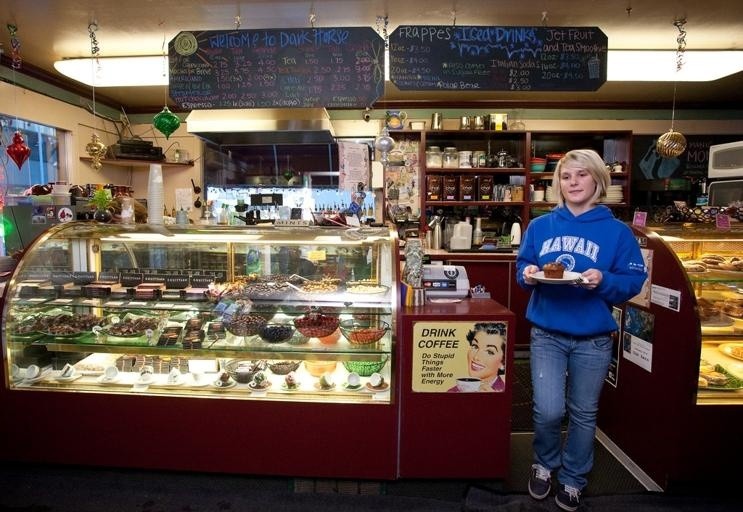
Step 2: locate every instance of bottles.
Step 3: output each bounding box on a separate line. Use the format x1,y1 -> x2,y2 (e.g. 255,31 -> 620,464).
510,222 -> 520,245
473,216 -> 482,245
473,115 -> 484,130
459,116 -> 470,129
425,146 -> 518,168
46,181 -> 68,194
312,201 -> 373,218
429,113 -> 444,130
423,216 -> 443,250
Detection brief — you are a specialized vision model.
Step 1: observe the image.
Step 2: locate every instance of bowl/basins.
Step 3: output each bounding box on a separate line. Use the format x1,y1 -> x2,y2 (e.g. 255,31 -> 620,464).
219,313 -> 389,384
673,200 -> 687,211
529,153 -> 566,172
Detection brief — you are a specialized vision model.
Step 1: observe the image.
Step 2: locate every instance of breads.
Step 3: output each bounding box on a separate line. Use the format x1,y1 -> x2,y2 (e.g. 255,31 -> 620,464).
696,297 -> 743,326
681,254 -> 743,272
692,281 -> 729,290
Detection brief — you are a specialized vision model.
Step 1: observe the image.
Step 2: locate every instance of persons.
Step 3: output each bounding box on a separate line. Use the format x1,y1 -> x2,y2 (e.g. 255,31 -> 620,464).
446,322 -> 506,392
348,192 -> 366,220
515,150 -> 648,511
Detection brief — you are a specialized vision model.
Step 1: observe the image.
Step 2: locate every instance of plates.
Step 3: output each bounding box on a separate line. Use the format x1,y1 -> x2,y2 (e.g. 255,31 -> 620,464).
545,185 -> 554,202
98,371 -> 210,389
7,313 -> 157,338
340,381 -> 365,391
213,380 -> 236,389
281,382 -> 302,392
342,283 -> 388,298
365,382 -> 389,393
313,382 -> 338,392
23,372 -> 85,386
529,271 -> 584,284
248,380 -> 272,390
600,184 -> 624,204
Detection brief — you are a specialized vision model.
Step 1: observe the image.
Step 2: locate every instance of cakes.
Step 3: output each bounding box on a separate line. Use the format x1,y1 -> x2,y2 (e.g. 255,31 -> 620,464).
542,263 -> 566,278
698,359 -> 727,388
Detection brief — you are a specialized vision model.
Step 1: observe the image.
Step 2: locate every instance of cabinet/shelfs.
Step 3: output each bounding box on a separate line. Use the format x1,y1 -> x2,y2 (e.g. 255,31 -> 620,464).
0,220 -> 403,485
397,252 -> 534,362
591,219 -> 743,494
375,126 -> 634,255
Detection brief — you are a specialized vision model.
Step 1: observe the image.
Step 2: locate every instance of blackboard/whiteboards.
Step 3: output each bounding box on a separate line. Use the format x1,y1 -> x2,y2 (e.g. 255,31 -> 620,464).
169,28 -> 385,112
389,26 -> 608,92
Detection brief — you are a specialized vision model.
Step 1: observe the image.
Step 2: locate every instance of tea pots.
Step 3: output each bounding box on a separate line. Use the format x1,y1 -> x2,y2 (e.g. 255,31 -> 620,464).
384,110 -> 406,130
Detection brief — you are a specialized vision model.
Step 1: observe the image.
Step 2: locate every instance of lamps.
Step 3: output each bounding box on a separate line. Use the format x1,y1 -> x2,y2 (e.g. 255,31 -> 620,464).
147,21 -> 182,141
6,19 -> 31,172
76,19 -> 108,173
656,16 -> 692,160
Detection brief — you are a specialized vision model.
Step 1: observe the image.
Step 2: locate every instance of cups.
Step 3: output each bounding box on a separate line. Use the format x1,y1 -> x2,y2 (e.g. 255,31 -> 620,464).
216,212 -> 245,225
532,190 -> 545,202
146,164 -> 164,225
454,378 -> 491,392
121,198 -> 135,224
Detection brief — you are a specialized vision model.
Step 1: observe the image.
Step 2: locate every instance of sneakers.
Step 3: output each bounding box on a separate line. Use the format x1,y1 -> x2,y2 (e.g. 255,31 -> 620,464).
554,484 -> 583,512
527,464 -> 552,500
